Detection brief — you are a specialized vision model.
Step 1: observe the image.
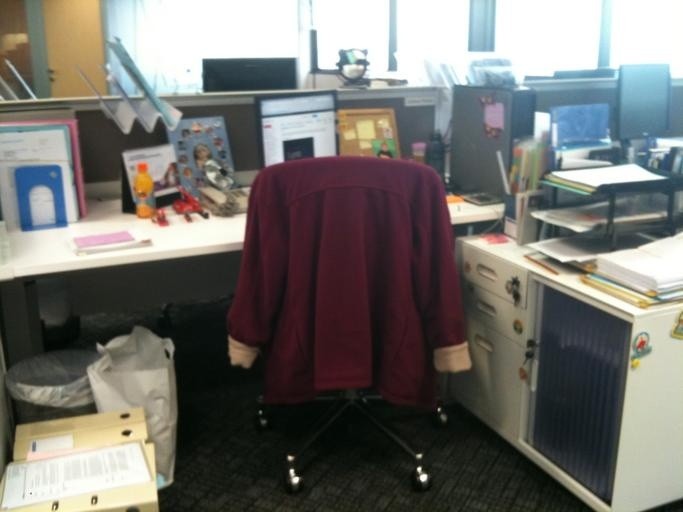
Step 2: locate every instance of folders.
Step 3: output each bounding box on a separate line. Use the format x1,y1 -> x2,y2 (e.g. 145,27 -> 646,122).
1,406 -> 159,512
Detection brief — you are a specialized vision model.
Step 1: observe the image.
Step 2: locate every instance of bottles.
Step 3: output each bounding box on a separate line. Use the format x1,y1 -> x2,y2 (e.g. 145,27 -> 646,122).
133,162 -> 156,219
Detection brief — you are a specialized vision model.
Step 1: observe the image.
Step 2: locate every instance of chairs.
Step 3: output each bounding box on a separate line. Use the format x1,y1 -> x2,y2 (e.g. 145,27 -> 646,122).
247,154 -> 453,496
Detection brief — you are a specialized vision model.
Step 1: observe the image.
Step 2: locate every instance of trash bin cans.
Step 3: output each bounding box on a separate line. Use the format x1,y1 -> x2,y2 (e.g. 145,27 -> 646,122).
6,350 -> 97,426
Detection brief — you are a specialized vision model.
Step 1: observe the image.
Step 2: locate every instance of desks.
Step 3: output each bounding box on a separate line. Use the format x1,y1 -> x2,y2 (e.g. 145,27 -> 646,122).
2,191 -> 511,386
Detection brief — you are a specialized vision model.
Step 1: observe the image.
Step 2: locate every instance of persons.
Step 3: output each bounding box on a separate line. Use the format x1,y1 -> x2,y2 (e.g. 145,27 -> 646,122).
191,144 -> 212,169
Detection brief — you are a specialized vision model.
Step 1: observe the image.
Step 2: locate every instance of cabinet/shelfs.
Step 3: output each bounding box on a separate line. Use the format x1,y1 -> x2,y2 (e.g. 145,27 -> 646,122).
445,234 -> 683,512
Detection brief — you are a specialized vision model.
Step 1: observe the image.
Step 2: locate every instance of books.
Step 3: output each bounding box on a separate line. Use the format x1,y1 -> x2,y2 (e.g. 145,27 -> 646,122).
541,163 -> 669,196
584,231 -> 682,309
0,118 -> 87,234
71,228 -> 151,258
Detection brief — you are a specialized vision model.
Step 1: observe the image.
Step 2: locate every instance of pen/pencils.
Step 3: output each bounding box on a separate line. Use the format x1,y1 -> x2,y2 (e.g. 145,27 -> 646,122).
199,210 -> 210,219
182,210 -> 192,224
524,254 -> 560,275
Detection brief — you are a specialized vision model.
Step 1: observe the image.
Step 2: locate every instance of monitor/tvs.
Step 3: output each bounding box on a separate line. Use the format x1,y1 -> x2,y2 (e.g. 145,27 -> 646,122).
202,58 -> 297,91
254,90 -> 339,168
